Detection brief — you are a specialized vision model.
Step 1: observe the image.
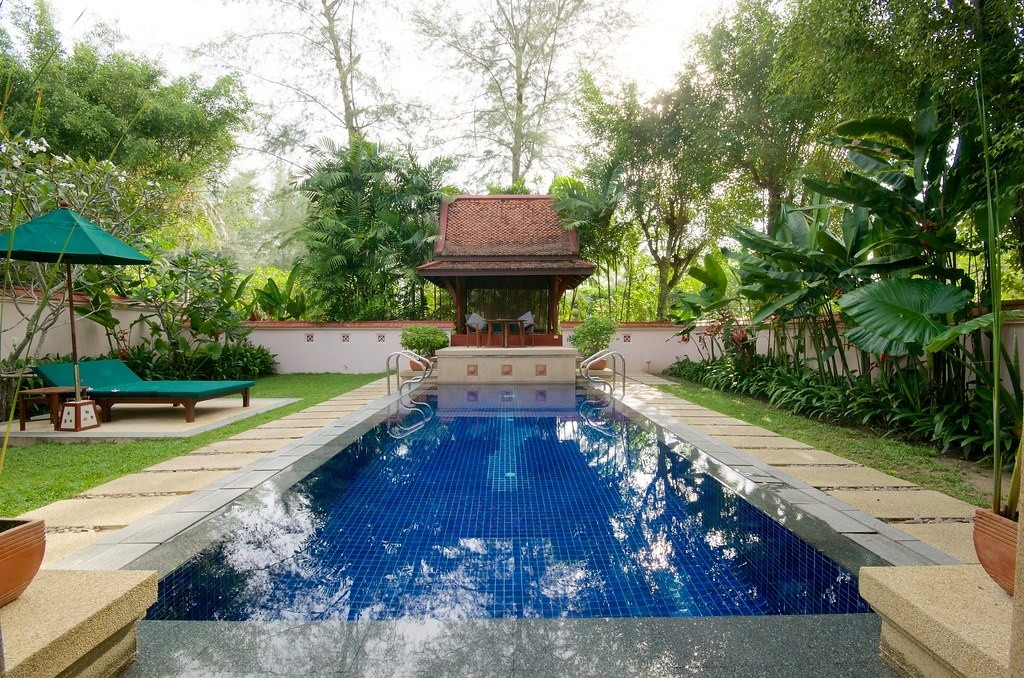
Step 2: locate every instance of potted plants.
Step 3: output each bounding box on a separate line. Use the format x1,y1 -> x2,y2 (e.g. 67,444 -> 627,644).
975,48 -> 1024,600
568,310 -> 618,370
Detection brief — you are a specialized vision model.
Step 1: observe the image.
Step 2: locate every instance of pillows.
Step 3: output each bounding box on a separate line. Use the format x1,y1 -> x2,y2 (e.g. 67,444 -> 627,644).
516,310 -> 534,329
467,313 -> 486,332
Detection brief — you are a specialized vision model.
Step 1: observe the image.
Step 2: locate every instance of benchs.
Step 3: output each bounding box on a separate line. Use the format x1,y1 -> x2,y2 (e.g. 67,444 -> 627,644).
33,359 -> 256,422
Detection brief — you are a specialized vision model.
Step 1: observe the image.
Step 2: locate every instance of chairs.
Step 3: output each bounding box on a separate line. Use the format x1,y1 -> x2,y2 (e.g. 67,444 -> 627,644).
518,315 -> 536,346
465,314 -> 497,348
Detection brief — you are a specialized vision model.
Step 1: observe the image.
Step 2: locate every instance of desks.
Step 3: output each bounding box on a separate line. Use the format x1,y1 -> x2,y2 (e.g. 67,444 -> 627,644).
19,387 -> 88,431
486,319 -> 528,347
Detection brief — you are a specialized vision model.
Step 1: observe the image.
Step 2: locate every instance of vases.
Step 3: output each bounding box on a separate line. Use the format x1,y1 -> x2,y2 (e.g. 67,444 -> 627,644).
0,518 -> 46,607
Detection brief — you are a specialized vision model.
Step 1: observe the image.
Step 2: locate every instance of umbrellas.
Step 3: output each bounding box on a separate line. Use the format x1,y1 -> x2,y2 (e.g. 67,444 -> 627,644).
0,199 -> 152,401
